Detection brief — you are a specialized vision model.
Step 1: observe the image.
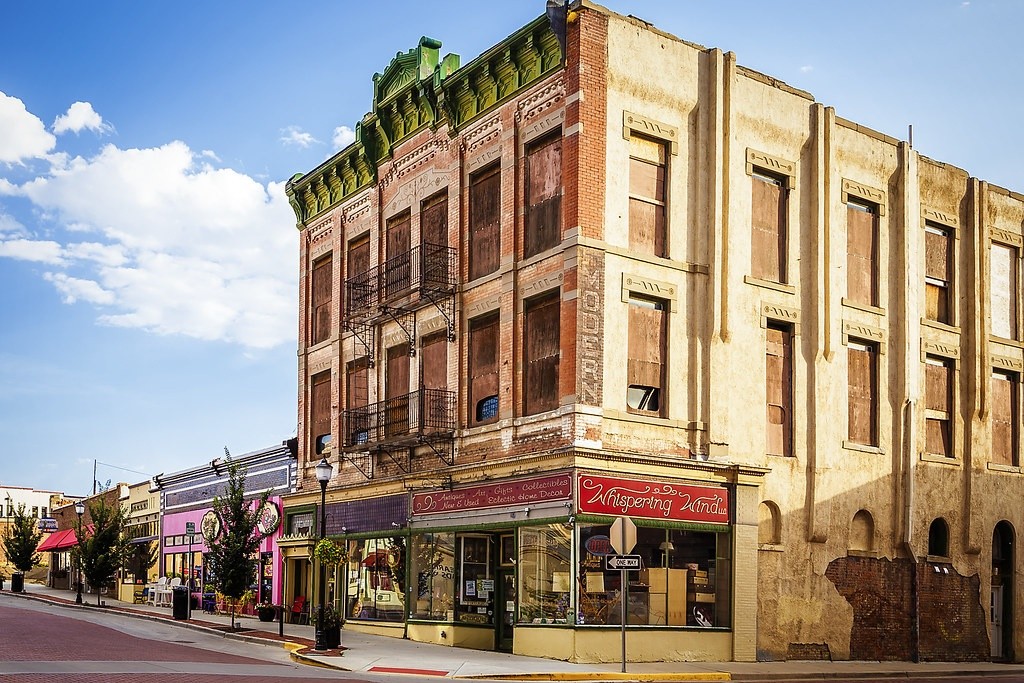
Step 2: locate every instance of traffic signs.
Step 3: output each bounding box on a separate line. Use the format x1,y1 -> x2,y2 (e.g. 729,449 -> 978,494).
606,554 -> 641,570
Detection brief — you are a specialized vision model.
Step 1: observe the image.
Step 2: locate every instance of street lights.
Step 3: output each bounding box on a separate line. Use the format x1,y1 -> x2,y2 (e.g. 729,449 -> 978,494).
315,454 -> 333,650
75,499 -> 85,604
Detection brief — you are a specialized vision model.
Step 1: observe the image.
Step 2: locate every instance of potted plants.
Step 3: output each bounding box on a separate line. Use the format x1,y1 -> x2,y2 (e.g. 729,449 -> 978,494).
310,606 -> 345,650
254,603 -> 275,621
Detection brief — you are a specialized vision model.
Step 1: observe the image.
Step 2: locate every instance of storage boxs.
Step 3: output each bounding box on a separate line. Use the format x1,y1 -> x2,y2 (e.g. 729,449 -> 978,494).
586,572 -> 604,592
639,563 -> 716,626
552,572 -> 569,592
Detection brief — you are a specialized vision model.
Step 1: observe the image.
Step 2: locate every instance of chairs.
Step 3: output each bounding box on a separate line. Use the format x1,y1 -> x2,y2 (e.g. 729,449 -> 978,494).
297,601 -> 310,624
288,596 -> 305,624
133,576 -> 182,608
